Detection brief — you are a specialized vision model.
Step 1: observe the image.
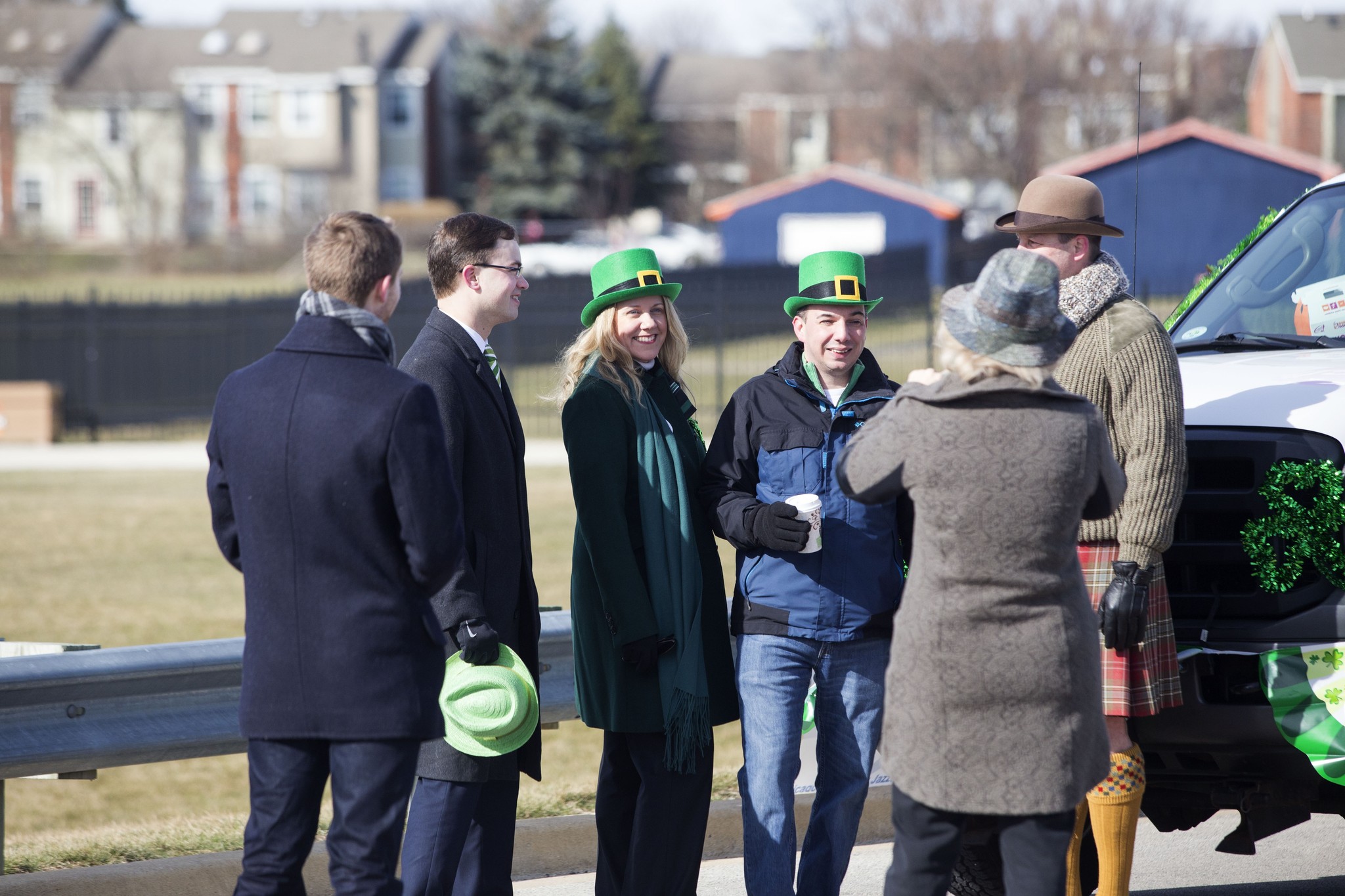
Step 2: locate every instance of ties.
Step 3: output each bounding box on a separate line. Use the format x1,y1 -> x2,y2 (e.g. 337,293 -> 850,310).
483,345 -> 502,389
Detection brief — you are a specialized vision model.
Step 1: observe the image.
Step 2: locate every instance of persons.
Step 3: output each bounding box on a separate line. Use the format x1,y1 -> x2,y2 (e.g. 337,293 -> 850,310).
560,245 -> 740,896
839,168 -> 1186,896
202,211 -> 457,896
696,250 -> 913,896
396,213 -> 542,895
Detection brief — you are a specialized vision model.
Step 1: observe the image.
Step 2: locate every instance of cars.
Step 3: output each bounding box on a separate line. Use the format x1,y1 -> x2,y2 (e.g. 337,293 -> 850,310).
1153,176 -> 1345,763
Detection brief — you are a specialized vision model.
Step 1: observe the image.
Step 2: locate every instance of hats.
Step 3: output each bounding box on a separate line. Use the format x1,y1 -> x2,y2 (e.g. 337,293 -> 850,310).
783,251 -> 883,320
940,248 -> 1076,366
437,643 -> 539,758
581,248 -> 683,329
993,175 -> 1124,238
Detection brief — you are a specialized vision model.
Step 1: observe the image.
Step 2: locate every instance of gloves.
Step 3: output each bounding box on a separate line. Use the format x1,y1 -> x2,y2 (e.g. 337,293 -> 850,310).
454,618 -> 499,665
1099,561 -> 1154,652
745,501 -> 811,551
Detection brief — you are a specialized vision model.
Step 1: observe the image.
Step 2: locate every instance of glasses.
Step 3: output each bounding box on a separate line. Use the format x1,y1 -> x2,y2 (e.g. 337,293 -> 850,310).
460,264 -> 523,278
